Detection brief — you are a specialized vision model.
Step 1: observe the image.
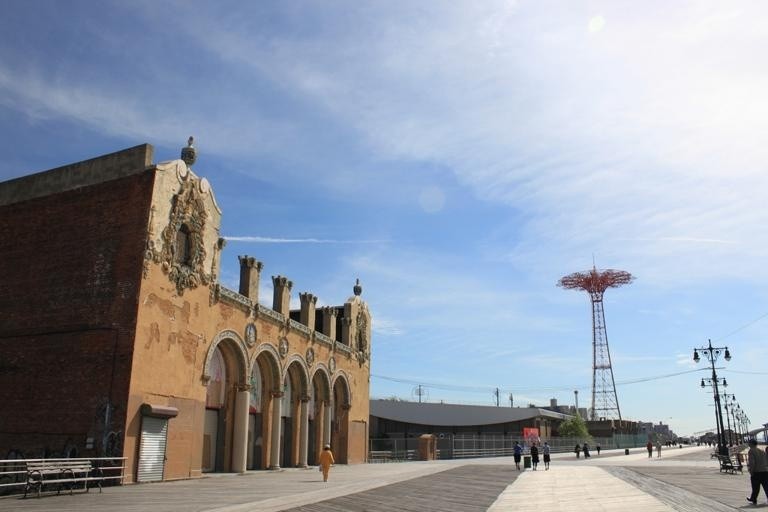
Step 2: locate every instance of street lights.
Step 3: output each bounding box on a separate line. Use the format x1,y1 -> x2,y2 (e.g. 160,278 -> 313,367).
700,376 -> 751,446
692,338 -> 731,446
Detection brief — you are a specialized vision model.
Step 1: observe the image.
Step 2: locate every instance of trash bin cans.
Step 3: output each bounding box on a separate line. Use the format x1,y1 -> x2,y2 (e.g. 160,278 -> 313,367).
625,449 -> 629,455
679,445 -> 682,448
524,457 -> 531,468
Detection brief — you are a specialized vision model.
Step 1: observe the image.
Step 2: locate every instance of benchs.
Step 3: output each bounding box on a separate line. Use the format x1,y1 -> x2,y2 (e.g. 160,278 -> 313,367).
717,454 -> 743,474
22,461 -> 105,498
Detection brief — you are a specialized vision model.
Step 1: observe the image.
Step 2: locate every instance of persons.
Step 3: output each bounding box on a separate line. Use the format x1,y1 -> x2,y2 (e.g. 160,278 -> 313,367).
746,439 -> 768,504
574,437 -> 720,458
543,441 -> 551,469
531,441 -> 539,470
513,442 -> 522,468
319,444 -> 334,481
